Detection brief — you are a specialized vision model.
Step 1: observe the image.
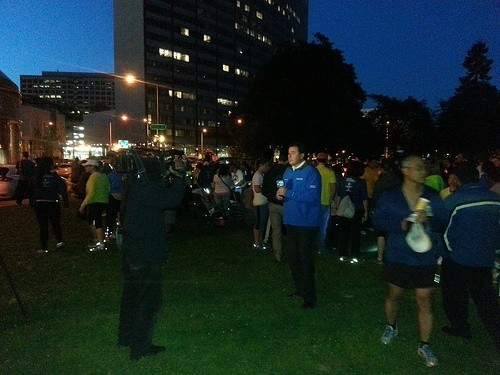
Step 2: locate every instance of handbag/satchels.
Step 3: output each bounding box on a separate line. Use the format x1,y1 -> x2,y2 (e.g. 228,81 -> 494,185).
329,195 -> 355,217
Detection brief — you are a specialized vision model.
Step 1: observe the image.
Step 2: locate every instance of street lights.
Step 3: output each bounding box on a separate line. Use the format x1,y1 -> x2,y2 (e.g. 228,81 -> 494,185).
122,115 -> 149,149
126,74 -> 159,150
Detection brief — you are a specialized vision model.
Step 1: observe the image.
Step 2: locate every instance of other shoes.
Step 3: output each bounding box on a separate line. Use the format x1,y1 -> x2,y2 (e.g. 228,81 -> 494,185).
338,256 -> 350,262
37,249 -> 49,254
252,242 -> 266,250
55,241 -> 65,248
288,291 -> 304,298
349,258 -> 365,264
381,322 -> 399,344
375,258 -> 384,264
442,326 -> 472,340
416,341 -> 440,368
88,243 -> 108,251
300,301 -> 314,309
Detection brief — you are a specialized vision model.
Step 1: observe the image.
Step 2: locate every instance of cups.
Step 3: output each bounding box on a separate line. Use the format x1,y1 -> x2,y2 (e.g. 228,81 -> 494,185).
414,197 -> 430,212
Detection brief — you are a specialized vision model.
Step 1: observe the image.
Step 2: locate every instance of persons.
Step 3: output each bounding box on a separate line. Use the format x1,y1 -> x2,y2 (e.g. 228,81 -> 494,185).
431,160 -> 500,350
367,150 -> 443,369
275,140 -> 324,311
10,143 -> 500,278
116,158 -> 190,361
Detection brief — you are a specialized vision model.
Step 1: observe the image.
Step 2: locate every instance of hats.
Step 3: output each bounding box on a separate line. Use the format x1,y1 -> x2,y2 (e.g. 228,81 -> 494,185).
404,221 -> 433,253
81,160 -> 99,167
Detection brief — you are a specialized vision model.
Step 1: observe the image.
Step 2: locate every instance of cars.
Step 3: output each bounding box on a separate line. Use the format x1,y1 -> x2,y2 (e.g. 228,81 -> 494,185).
0,164 -> 20,200
55,163 -> 71,178
